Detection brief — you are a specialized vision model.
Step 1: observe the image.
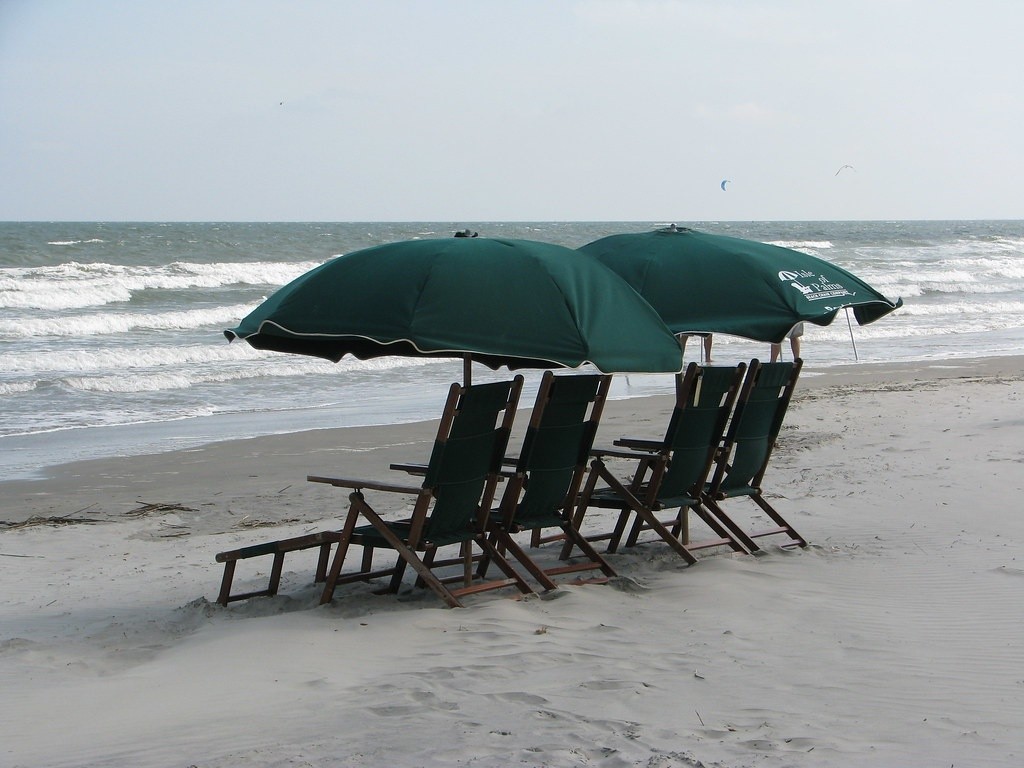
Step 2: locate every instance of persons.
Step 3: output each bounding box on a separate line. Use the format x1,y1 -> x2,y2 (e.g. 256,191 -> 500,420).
676,332 -> 713,362
770,322 -> 804,363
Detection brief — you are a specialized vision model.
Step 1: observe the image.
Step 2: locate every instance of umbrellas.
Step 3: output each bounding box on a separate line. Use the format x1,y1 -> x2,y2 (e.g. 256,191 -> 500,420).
225,235 -> 682,589
572,223 -> 899,546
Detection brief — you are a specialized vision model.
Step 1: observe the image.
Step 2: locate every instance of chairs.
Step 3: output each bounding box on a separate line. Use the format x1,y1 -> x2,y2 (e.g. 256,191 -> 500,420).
531,357 -> 808,565
307,370 -> 616,608
